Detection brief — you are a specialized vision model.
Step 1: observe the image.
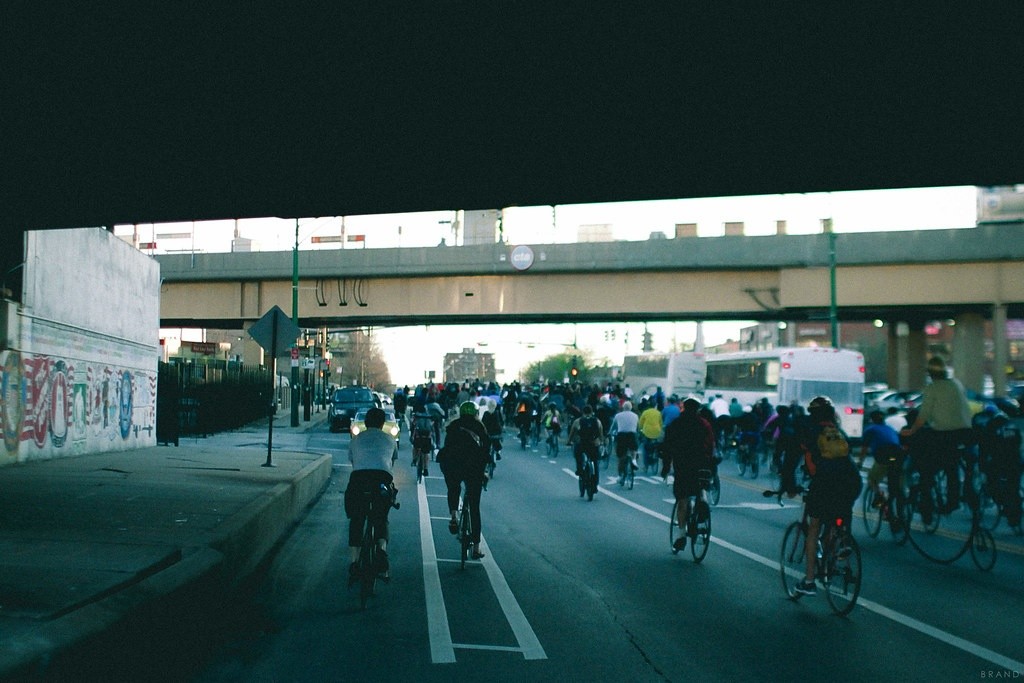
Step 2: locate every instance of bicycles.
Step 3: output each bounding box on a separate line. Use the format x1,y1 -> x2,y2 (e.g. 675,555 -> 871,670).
857,448 -> 912,547
765,482 -> 865,618
340,482 -> 400,609
975,464 -> 1023,538
392,394 -> 955,508
899,422 -> 999,574
659,458 -> 714,560
451,453 -> 497,569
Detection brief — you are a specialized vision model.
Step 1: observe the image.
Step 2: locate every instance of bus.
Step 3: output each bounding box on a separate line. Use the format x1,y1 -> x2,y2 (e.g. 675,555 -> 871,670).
616,352 -> 706,409
703,344 -> 865,441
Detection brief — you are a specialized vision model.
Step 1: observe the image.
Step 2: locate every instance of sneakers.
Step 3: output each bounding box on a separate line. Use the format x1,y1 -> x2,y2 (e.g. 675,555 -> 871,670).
795,580 -> 816,595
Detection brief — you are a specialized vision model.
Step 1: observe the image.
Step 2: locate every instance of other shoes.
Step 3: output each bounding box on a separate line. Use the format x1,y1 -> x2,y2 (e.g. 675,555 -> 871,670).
424,469 -> 428,476
375,550 -> 389,572
413,460 -> 416,466
349,562 -> 358,576
496,454 -> 501,460
473,551 -> 484,559
575,470 -> 584,476
449,519 -> 459,535
631,460 -> 638,469
616,476 -> 623,482
673,537 -> 686,549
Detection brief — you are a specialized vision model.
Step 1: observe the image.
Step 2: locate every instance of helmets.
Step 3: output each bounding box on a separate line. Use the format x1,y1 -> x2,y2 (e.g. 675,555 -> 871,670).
808,396 -> 833,413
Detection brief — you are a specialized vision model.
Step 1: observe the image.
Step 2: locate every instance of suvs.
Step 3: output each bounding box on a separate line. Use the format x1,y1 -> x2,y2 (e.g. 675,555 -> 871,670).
328,386 -> 378,430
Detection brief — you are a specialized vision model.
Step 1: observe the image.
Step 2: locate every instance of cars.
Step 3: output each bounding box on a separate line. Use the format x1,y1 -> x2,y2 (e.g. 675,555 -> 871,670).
371,392 -> 394,413
351,412 -> 401,451
858,381 -> 1024,422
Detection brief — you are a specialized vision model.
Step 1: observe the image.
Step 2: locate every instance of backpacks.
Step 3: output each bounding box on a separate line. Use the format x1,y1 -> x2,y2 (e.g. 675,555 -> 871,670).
579,416 -> 600,442
550,415 -> 559,427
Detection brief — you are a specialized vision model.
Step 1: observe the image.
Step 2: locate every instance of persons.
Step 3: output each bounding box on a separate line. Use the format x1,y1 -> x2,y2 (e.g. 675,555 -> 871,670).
346,408 -> 400,592
394,357 -> 1024,647
